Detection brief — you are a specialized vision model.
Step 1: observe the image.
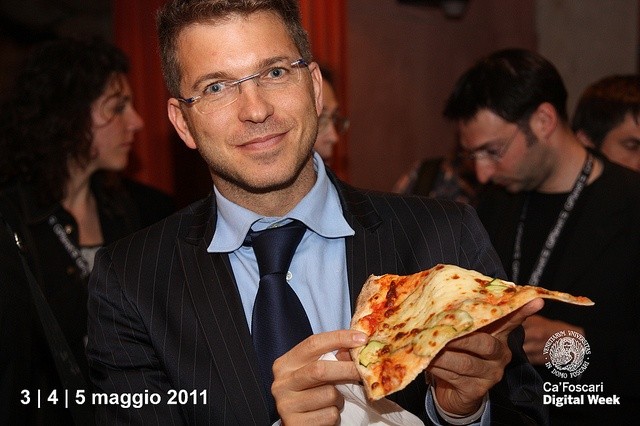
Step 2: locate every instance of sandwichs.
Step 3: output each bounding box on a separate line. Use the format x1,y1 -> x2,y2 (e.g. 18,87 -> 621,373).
343,261 -> 595,401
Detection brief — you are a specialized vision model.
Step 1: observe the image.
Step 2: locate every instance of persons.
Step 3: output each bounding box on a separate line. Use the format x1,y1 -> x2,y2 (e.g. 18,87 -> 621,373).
441,48 -> 639,423
570,77 -> 640,174
85,0 -> 544,425
312,70 -> 354,170
1,33 -> 174,424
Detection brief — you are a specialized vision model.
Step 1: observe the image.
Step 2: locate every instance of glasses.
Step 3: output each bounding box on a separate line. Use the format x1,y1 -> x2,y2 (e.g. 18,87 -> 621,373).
177,56 -> 311,104
461,123 -> 522,161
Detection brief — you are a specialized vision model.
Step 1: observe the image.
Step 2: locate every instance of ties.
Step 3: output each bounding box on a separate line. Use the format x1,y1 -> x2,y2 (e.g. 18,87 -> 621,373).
244,219 -> 315,424
